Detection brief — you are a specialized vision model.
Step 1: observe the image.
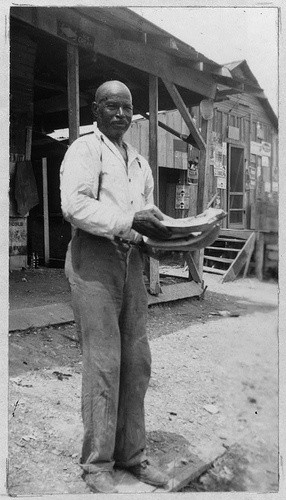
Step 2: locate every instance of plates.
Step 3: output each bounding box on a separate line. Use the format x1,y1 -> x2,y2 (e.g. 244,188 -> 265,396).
141,207 -> 227,247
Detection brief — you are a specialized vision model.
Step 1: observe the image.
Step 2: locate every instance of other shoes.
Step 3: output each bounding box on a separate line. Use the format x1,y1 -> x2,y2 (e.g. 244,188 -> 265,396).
77,464 -> 117,494
126,458 -> 169,486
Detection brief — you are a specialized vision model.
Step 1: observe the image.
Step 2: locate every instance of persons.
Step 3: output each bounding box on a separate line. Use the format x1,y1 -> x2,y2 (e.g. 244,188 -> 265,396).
56,79 -> 174,496
